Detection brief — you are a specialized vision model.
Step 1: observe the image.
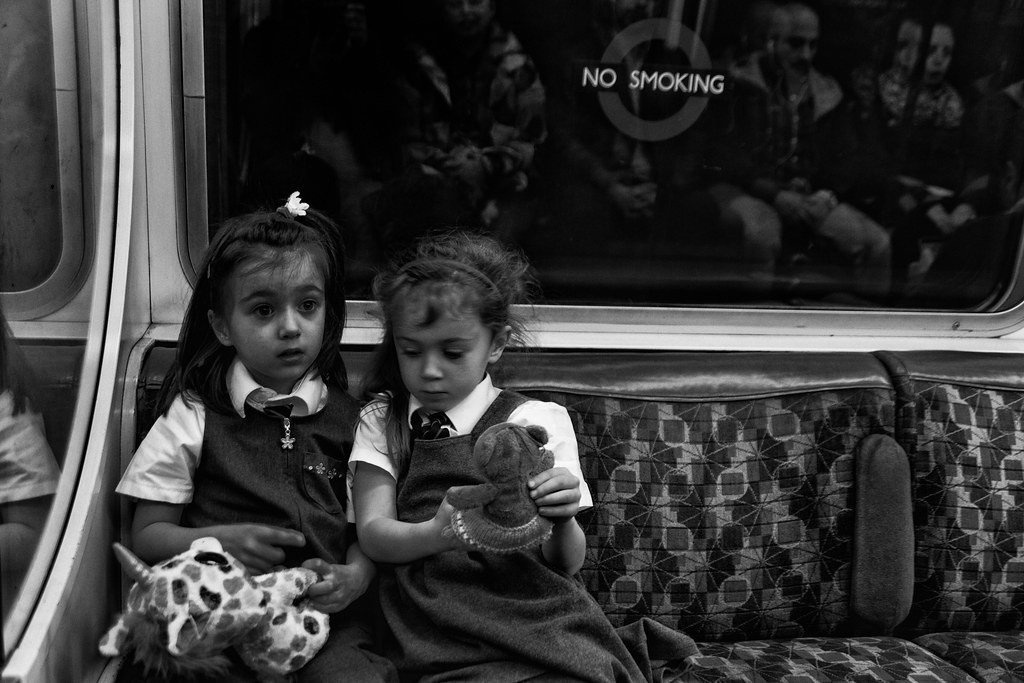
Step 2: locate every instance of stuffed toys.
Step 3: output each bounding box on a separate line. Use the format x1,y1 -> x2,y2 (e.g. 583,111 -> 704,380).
98,536 -> 330,676
440,422 -> 555,557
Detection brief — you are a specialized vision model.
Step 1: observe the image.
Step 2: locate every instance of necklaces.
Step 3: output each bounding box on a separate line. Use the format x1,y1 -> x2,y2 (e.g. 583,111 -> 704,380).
248,397 -> 297,449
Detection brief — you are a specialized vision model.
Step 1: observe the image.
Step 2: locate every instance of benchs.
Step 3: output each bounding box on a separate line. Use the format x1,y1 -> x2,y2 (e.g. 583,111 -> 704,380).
0,260 -> 1024,683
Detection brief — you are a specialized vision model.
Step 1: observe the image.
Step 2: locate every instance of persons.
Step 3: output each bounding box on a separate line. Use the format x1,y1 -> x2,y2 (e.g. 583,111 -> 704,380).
347,231 -> 700,682
0,310 -> 62,625
408,2 -> 975,304
115,191 -> 398,683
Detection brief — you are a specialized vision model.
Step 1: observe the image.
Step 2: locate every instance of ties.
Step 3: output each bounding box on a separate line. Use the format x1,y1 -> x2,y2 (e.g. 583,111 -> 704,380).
419,412 -> 452,440
264,402 -> 293,416
